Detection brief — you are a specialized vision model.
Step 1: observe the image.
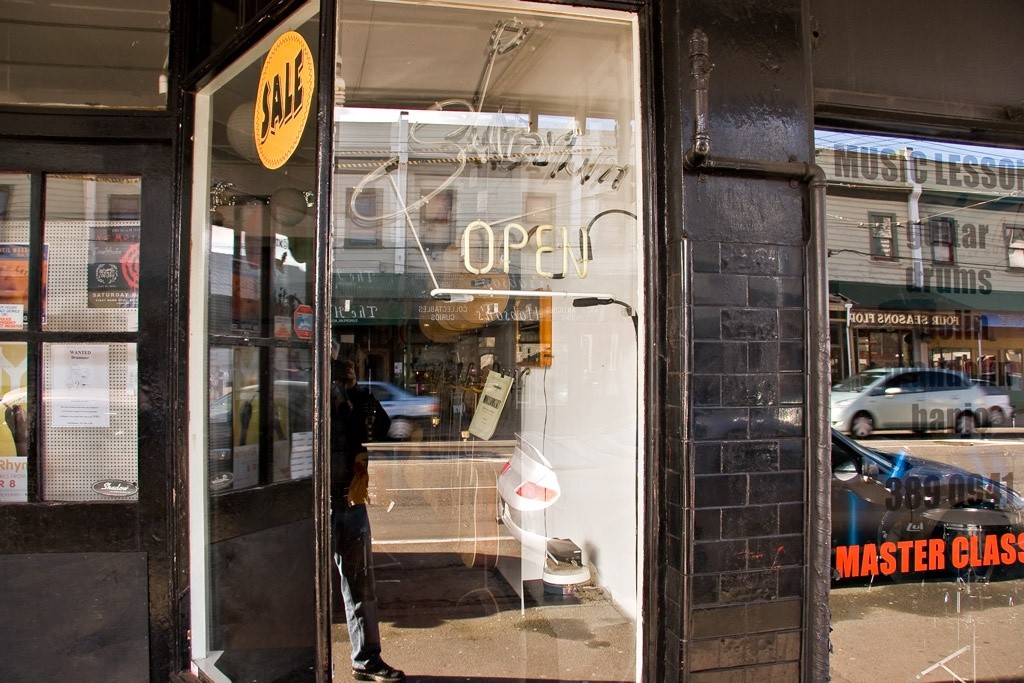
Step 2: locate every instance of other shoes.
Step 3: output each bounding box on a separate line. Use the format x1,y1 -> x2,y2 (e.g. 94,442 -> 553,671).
351,658 -> 406,683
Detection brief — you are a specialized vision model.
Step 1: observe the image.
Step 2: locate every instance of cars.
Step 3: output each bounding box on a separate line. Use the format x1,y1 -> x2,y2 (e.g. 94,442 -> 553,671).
970,378 -> 1014,426
830,363 -> 985,440
832,427 -> 1023,586
355,381 -> 442,443
209,380 -> 314,471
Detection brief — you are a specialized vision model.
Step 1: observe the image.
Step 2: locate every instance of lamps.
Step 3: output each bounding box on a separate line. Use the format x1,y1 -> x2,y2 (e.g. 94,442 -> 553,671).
573,297 -> 638,335
269,163 -> 307,226
227,56 -> 262,164
431,288 -> 614,304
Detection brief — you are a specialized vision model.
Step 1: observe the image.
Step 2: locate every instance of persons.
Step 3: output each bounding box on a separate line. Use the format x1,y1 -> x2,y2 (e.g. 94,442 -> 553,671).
329,338 -> 404,683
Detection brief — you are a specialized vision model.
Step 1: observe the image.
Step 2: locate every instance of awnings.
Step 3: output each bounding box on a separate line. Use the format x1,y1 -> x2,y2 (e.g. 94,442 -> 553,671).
830,280 -> 1024,312
